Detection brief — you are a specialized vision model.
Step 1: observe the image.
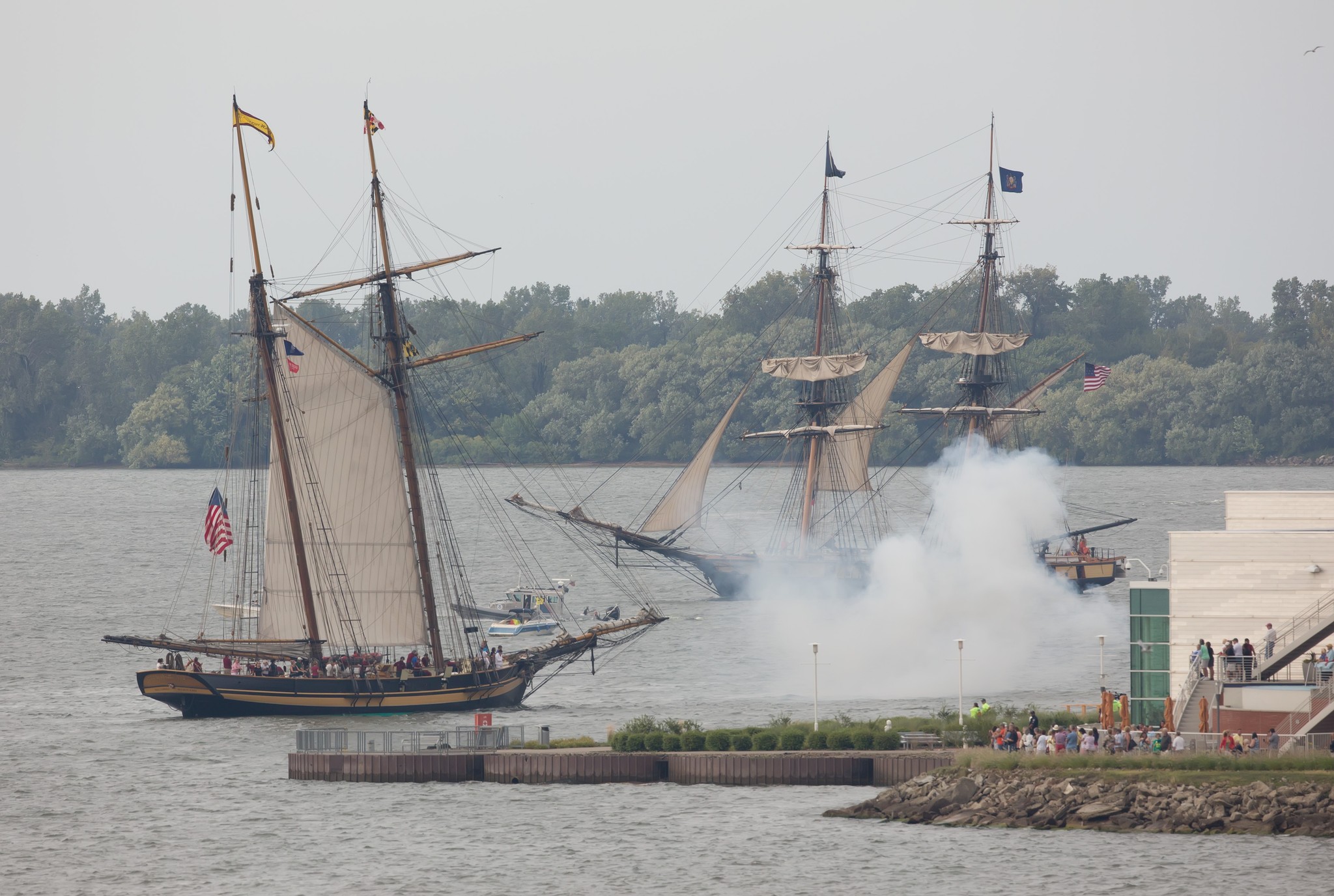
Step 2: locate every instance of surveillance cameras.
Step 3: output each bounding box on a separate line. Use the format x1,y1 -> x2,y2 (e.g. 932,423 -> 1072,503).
1125,562 -> 1131,570
1159,569 -> 1164,575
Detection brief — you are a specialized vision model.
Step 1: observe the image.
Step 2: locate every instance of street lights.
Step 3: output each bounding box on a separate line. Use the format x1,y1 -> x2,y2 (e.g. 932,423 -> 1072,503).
945,638 -> 976,725
1085,634 -> 1117,725
800,643 -> 831,730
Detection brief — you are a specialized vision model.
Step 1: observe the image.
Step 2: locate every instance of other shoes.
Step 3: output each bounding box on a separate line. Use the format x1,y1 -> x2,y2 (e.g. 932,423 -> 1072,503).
1223,680 -> 1230,683
1231,679 -> 1237,682
1203,678 -> 1209,680
1210,678 -> 1214,680
1199,676 -> 1205,680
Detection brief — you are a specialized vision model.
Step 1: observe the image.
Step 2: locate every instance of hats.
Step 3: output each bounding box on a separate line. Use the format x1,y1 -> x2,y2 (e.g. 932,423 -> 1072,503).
999,723 -> 1004,727
1130,724 -> 1137,730
981,699 -> 985,701
1245,639 -> 1249,642
1266,623 -> 1272,626
1052,724 -> 1058,730
1232,638 -> 1238,641
1002,722 -> 1007,727
1225,642 -> 1230,645
1326,644 -> 1332,647
1176,731 -> 1180,735
1030,711 -> 1034,714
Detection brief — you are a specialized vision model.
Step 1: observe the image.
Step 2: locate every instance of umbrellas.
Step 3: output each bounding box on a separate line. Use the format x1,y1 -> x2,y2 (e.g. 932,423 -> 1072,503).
1100,692 -> 1209,733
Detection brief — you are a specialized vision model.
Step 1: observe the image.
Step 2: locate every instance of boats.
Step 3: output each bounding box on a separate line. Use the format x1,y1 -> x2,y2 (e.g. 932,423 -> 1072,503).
486,596 -> 561,637
448,574 -> 601,622
212,586 -> 260,620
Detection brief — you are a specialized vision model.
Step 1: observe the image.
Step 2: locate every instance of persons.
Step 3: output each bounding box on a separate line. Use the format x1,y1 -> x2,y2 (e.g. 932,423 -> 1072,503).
1102,721 -> 1186,755
1317,644 -> 1334,662
1330,733 -> 1334,753
1218,728 -> 1279,756
1064,534 -> 1087,556
223,652 -> 377,678
970,699 -> 1099,753
193,657 -> 202,672
1113,699 -> 1120,712
477,642 -> 504,667
525,595 -> 531,608
156,658 -> 164,669
393,650 -> 431,677
1192,623 -> 1276,682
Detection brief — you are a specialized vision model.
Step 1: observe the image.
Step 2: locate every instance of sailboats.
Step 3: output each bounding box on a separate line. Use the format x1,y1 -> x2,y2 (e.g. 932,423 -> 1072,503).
502,104 -> 1141,604
100,88 -> 670,717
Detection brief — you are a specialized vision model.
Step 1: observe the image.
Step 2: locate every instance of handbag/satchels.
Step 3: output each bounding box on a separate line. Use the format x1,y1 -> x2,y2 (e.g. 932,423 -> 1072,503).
1128,739 -> 1137,749
1046,746 -> 1049,752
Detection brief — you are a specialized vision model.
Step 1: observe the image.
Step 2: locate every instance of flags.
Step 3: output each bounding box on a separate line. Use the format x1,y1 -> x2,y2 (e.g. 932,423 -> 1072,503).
282,339 -> 304,373
1084,362 -> 1111,392
826,140 -> 846,178
362,104 -> 385,135
999,166 -> 1023,193
205,487 -> 233,555
233,104 -> 275,152
